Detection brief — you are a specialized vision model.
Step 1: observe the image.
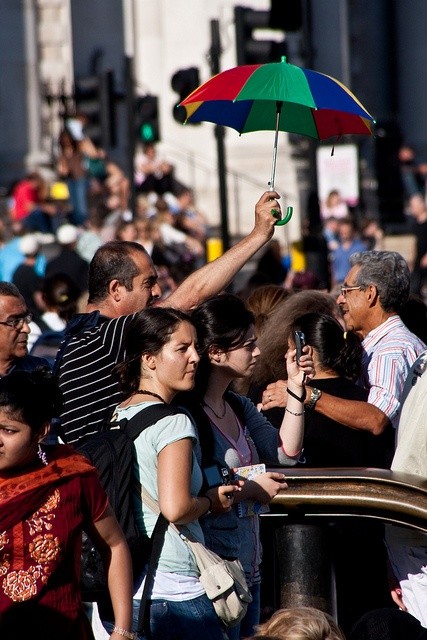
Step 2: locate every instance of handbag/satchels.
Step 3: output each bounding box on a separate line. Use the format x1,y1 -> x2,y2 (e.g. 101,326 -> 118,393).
187,539 -> 252,627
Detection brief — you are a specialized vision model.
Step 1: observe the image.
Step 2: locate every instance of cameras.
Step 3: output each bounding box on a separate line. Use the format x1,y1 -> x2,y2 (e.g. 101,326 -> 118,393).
220,468 -> 235,499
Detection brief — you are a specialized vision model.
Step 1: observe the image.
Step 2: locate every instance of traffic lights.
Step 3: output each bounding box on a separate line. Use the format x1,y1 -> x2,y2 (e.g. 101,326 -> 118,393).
77,69 -> 120,152
129,92 -> 160,146
233,0 -> 279,62
173,65 -> 201,129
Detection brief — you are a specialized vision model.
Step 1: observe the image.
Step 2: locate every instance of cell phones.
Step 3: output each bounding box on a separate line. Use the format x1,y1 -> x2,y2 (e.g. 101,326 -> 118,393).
296,330 -> 305,365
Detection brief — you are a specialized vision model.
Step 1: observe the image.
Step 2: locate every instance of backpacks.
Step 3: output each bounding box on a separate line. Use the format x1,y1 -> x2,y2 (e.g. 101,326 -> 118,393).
74,428 -> 148,602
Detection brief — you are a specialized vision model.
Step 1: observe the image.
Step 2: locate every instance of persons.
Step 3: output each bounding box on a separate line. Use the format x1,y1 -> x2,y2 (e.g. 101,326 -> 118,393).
9,234 -> 46,319
94,306 -> 244,639
259,316 -> 396,466
61,190 -> 281,451
0,282 -> 49,377
317,149 -> 426,281
187,294 -> 315,630
259,291 -> 350,377
1,370 -> 133,638
391,354 -> 427,627
176,57 -> 378,222
262,248 -> 426,437
246,285 -> 286,314
1,111 -> 204,260
22,254 -> 92,362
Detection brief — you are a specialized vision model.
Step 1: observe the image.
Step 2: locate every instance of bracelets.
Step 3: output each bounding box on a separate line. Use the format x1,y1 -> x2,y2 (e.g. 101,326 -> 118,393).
112,627 -> 138,639
282,406 -> 308,420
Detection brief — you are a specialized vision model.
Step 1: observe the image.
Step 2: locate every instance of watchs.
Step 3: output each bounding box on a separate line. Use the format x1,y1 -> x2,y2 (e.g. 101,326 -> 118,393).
306,383 -> 321,413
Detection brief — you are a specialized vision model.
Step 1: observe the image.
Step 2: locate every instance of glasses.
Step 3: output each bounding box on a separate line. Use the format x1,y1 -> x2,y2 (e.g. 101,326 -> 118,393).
340,286 -> 360,297
0,313 -> 32,329
